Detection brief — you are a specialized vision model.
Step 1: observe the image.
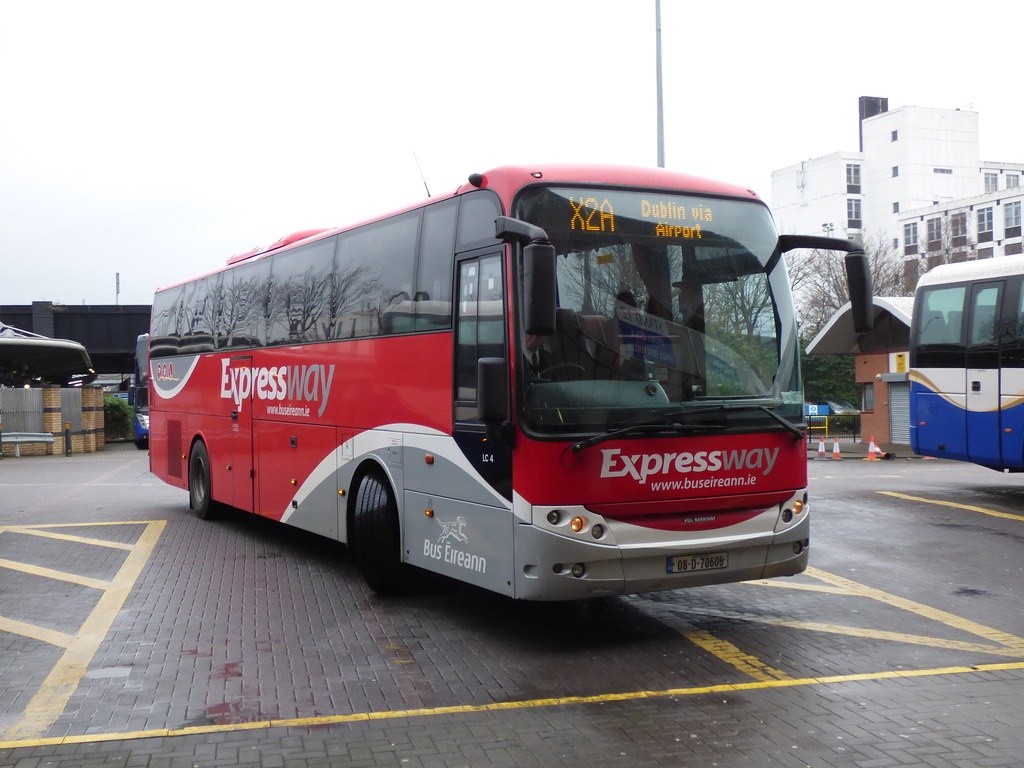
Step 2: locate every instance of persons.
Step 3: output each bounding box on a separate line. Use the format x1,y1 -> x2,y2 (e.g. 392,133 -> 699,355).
519,332 -> 564,398
600,289 -> 650,382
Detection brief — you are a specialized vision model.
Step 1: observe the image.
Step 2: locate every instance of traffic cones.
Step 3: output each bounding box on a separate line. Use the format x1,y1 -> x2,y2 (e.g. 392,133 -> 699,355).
872,444 -> 883,455
829,436 -> 843,461
862,434 -> 880,462
878,450 -> 895,461
813,437 -> 831,461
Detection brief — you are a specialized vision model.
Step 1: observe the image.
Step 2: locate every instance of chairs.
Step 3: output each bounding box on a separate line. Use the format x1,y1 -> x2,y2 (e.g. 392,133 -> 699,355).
152,297 -> 656,420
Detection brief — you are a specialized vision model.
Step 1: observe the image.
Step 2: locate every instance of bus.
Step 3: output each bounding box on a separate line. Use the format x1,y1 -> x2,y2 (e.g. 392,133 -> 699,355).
127,333 -> 155,451
147,161 -> 875,614
908,251 -> 1024,476
